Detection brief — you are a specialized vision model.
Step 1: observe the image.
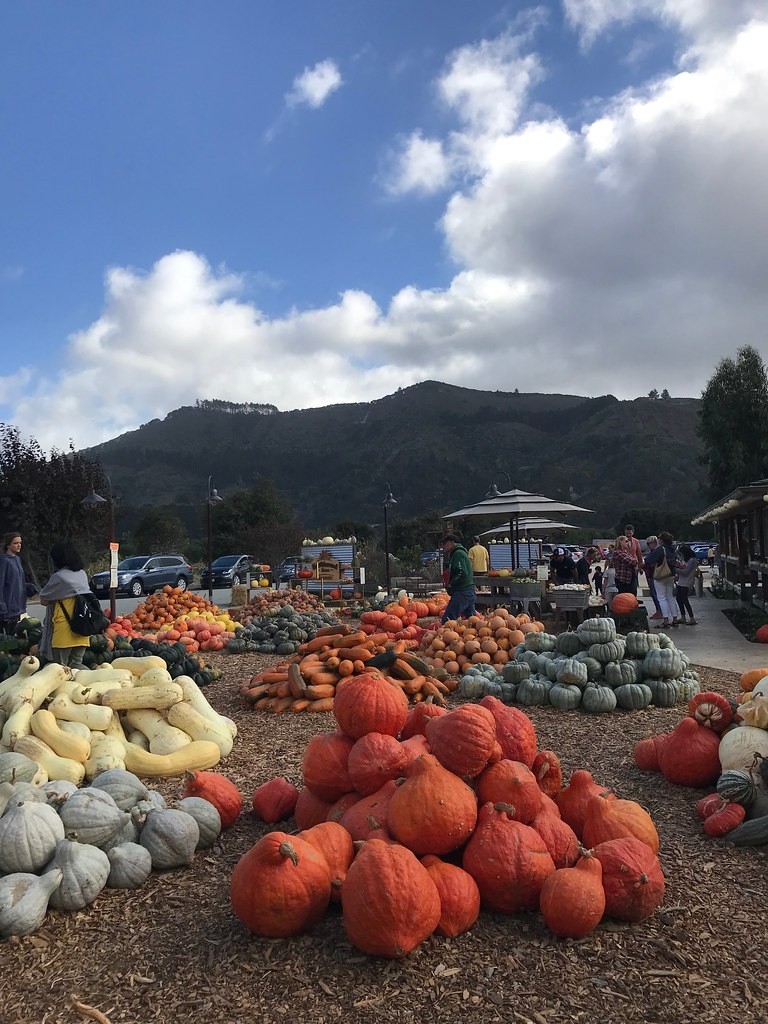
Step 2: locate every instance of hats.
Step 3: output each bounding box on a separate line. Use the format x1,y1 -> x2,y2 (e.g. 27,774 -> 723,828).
471,535 -> 481,543
553,547 -> 565,559
439,533 -> 463,544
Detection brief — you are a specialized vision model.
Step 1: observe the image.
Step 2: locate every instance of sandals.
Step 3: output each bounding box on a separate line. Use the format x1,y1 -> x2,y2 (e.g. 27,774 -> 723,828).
649,612 -> 663,620
653,622 -> 671,629
670,620 -> 679,627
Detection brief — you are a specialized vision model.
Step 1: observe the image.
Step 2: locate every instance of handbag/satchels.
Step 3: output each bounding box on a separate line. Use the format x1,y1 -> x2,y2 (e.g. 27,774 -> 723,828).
652,545 -> 672,580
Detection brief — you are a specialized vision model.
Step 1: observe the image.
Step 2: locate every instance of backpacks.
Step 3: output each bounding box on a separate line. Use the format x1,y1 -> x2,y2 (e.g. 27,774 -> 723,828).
57,592 -> 110,636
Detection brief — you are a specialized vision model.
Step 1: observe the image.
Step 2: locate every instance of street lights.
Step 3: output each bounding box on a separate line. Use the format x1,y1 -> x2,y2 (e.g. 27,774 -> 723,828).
205,475 -> 224,604
486,471 -> 516,571
80,471 -> 117,625
381,481 -> 399,596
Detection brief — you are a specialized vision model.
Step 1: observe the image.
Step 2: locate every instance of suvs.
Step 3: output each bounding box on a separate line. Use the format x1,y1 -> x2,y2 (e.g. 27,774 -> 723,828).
87,554 -> 194,598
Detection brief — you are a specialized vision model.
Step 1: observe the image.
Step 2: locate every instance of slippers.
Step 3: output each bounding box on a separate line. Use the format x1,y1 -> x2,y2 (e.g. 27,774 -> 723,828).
686,619 -> 698,625
676,620 -> 687,624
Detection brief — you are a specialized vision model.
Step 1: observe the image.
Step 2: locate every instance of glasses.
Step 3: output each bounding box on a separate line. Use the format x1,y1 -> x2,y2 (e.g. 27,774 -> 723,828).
646,541 -> 653,546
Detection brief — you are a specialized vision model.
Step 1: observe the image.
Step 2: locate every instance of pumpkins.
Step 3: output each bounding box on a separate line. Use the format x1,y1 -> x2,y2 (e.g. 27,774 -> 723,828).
0,535 -> 768,957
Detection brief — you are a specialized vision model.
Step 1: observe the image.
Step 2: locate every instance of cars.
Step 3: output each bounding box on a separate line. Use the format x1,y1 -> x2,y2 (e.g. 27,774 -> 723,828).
540,543 -> 609,565
276,555 -> 301,581
673,541 -> 721,566
418,548 -> 444,567
200,555 -> 263,589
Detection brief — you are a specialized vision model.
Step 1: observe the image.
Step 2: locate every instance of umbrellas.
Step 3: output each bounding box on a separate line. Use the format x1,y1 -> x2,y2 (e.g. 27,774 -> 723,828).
442,486 -> 594,572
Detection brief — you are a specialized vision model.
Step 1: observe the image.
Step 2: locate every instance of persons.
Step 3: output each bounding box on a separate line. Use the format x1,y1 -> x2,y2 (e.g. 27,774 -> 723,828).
438,535 -> 477,625
548,523 -> 722,629
0,532 -> 39,635
39,542 -> 92,671
467,534 -> 490,592
